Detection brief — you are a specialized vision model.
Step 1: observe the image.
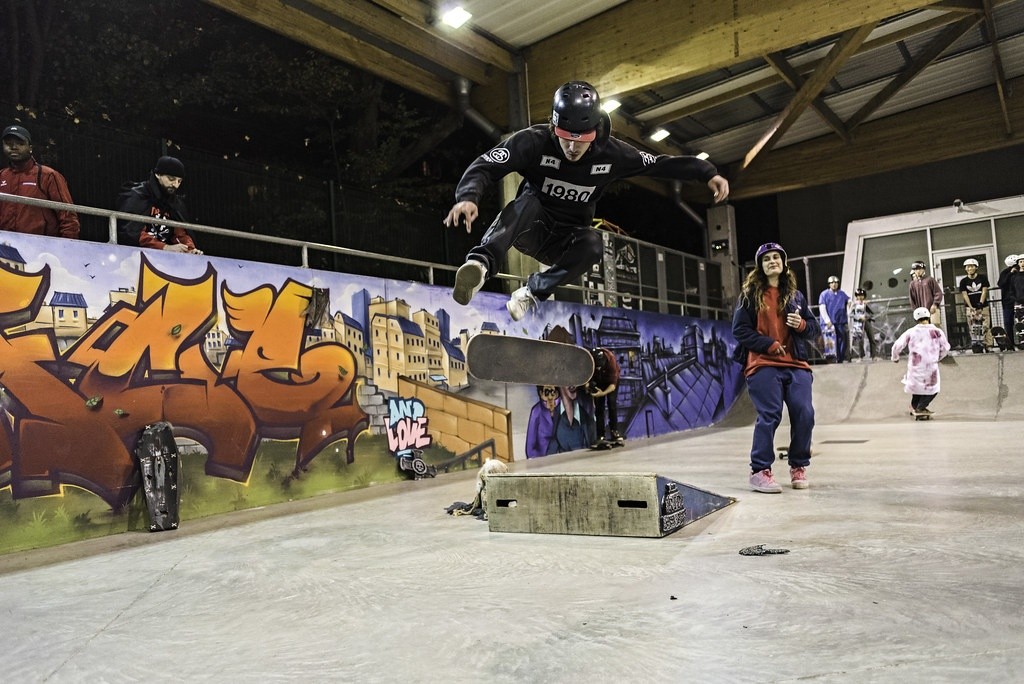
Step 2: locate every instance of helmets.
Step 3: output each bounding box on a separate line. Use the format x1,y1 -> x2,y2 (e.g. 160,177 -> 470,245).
910,269 -> 915,276
590,348 -> 605,366
855,288 -> 866,299
1016,254 -> 1024,264
912,261 -> 926,270
828,276 -> 840,284
912,306 -> 930,321
1004,255 -> 1018,266
963,259 -> 979,267
755,243 -> 787,268
552,80 -> 602,133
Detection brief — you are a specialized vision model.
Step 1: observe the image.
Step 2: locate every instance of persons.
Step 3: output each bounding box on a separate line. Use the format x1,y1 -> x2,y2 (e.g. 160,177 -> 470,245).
819,276 -> 850,363
960,258 -> 995,353
0,125 -> 80,239
849,287 -> 881,363
909,261 -> 943,314
731,242 -> 821,492
997,253 -> 1024,352
443,81 -> 729,323
587,347 -> 623,444
891,307 -> 951,413
116,156 -> 204,255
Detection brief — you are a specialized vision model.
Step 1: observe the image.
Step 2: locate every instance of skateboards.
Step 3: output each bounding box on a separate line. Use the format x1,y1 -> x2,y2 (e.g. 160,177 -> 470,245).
909,403 -> 934,422
991,326 -> 1015,352
777,447 -> 789,460
930,309 -> 940,328
970,307 -> 986,353
1014,301 -> 1024,345
590,437 -> 625,451
851,300 -> 865,342
822,325 -> 838,363
466,334 -> 593,387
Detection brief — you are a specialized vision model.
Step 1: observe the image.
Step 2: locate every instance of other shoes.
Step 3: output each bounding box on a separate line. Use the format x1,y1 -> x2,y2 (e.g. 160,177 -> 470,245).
856,358 -> 863,363
915,408 -> 930,413
872,357 -> 882,361
986,347 -> 994,353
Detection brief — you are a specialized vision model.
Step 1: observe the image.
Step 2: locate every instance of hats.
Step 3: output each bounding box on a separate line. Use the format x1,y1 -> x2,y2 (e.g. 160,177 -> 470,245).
555,126 -> 596,141
154,156 -> 185,178
2,125 -> 32,145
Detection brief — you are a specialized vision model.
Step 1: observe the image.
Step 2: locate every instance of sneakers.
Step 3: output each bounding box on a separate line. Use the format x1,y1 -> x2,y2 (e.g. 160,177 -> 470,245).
610,430 -> 623,440
506,286 -> 539,321
790,466 -> 809,488
749,469 -> 782,493
453,260 -> 487,305
597,436 -> 606,441
